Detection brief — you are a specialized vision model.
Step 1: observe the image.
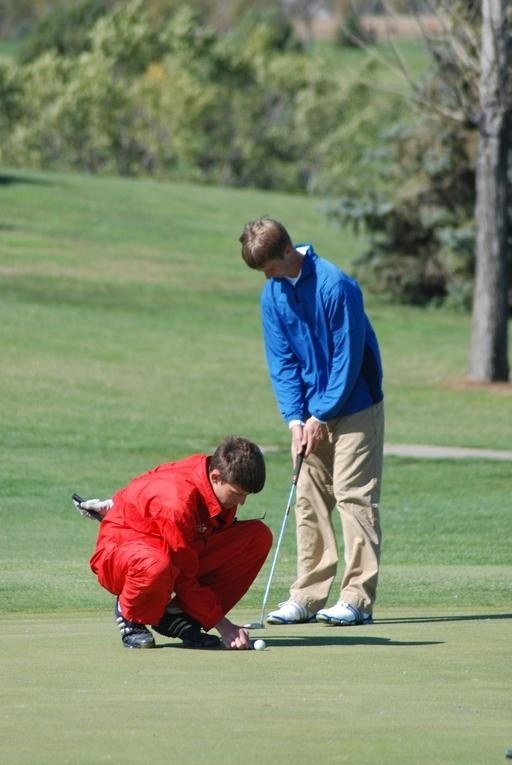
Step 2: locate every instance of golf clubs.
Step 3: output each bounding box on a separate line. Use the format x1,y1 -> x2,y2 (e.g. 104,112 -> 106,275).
241,445 -> 306,629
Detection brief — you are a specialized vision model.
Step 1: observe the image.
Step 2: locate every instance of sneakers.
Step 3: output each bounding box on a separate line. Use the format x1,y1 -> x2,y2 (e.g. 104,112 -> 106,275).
150,609 -> 220,648
114,596 -> 156,648
265,595 -> 316,625
316,600 -> 373,626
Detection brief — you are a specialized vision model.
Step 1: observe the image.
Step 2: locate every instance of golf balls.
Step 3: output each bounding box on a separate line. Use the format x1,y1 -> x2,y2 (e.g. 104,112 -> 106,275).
254,640 -> 265,650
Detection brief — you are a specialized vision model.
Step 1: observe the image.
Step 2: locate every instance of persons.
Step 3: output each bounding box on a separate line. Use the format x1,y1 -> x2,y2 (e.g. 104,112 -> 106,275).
73,434 -> 274,652
238,214 -> 387,625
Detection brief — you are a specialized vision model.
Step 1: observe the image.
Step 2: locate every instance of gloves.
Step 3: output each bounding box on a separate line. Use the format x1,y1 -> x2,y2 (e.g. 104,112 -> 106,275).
72,497 -> 114,521
